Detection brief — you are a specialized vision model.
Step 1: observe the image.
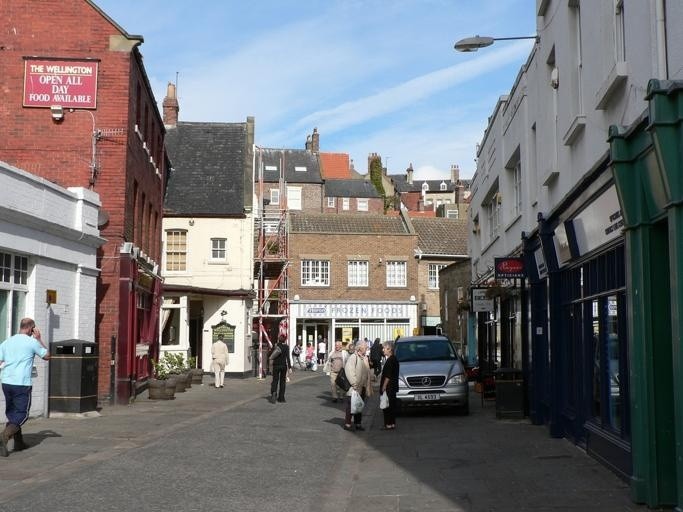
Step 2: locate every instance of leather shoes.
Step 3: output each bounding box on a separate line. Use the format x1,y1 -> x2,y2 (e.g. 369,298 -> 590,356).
345,426 -> 365,432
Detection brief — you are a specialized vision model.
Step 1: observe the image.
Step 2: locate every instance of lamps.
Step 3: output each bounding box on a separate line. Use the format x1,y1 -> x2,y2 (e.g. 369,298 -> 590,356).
49,104 -> 65,122
450,30 -> 542,55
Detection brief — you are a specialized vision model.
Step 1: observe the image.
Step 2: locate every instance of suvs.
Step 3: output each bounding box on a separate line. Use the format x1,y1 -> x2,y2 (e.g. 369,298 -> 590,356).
593,331 -> 623,410
391,334 -> 470,416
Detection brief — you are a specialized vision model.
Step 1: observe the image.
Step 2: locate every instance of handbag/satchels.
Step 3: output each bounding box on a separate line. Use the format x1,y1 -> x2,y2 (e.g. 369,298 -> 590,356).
269,343 -> 281,359
335,368 -> 351,391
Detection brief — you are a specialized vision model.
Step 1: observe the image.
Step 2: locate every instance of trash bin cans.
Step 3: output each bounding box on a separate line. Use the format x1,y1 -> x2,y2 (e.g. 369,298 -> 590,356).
493,367 -> 525,420
48,339 -> 99,412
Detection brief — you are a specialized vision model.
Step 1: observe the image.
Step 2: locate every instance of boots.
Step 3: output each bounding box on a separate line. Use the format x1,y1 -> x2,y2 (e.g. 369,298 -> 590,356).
0,423 -> 28,457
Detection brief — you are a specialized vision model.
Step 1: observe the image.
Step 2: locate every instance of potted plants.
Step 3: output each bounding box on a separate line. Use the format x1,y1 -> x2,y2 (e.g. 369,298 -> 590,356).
144,344 -> 204,402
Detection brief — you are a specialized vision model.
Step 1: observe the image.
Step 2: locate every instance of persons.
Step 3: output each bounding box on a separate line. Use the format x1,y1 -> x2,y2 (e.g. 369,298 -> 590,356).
379,341 -> 399,431
0,318 -> 51,457
344,340 -> 370,432
211,334 -> 229,388
269,334 -> 291,404
370,338 -> 385,380
323,341 -> 350,403
292,334 -> 373,371
